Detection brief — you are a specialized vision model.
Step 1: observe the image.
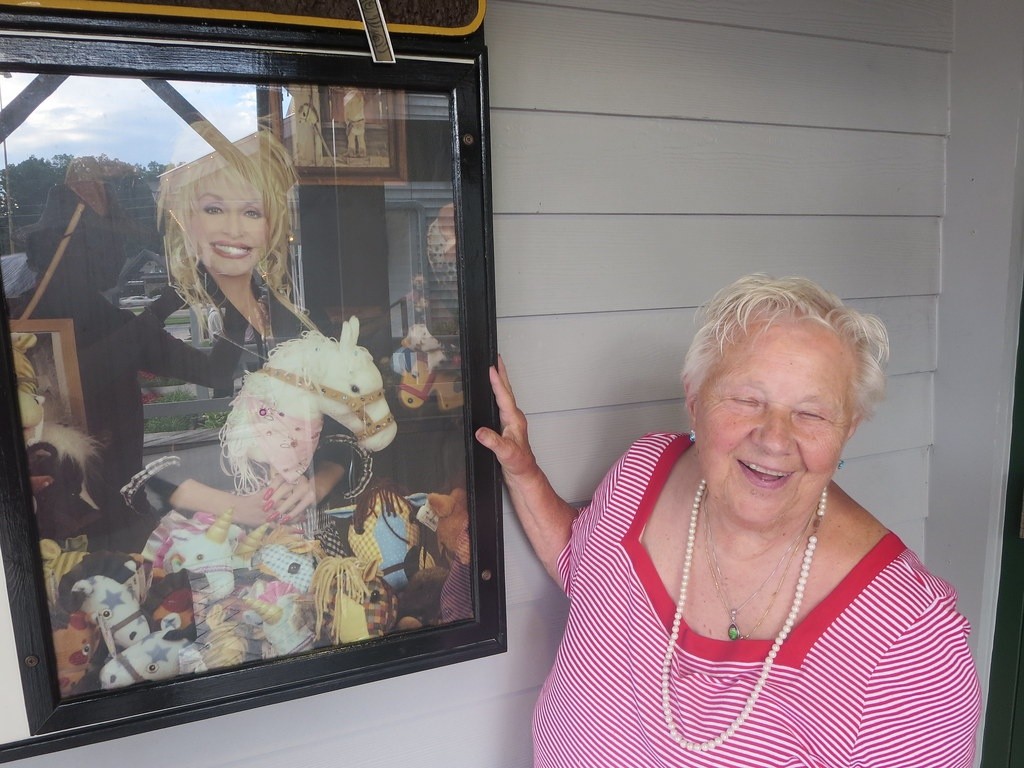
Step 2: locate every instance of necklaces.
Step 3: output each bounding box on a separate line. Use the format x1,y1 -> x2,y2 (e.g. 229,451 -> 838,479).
662,477 -> 828,752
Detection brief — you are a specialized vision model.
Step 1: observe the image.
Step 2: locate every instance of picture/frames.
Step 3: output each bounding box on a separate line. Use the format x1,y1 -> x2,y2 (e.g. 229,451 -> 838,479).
255,83 -> 409,187
0,12 -> 508,764
10,317 -> 91,436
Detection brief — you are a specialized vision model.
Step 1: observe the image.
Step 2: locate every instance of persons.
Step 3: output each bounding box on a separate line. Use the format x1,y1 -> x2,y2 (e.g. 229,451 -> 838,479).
475,272 -> 982,768
73,121 -> 375,583
